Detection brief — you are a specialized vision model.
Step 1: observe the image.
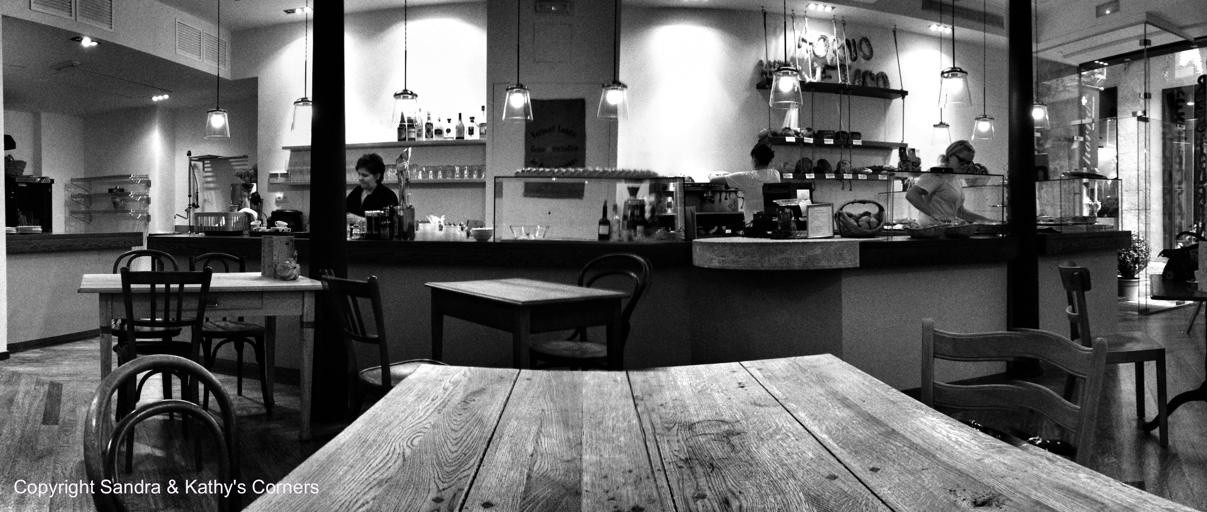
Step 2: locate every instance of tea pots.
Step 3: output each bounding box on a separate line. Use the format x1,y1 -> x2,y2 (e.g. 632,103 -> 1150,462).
274,259 -> 300,281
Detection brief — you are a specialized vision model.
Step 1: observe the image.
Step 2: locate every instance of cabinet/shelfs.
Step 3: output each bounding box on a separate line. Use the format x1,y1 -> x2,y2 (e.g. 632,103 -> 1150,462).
266,138 -> 488,194
754,57 -> 909,184
69,172 -> 152,225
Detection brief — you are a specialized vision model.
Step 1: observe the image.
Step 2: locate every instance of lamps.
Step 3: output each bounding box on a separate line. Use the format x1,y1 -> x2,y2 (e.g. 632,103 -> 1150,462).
201,0 -> 1052,143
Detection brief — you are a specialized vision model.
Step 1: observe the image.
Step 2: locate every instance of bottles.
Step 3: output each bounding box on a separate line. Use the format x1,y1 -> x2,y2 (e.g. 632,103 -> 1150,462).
598,185 -> 649,240
406,192 -> 415,229
418,220 -> 467,241
397,104 -> 488,141
365,210 -> 382,234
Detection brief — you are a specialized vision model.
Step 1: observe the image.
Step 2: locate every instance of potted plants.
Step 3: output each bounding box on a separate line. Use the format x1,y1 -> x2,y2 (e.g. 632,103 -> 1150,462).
1118,231 -> 1154,303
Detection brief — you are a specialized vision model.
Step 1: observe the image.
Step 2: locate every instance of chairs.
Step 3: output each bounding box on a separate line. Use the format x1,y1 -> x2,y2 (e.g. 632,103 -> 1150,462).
69,247 -> 451,509
917,318 -> 1110,469
526,249 -> 650,370
1055,251 -> 1172,446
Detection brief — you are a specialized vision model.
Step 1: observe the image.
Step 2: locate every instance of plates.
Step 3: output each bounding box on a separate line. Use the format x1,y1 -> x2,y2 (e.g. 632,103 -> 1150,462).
6,225 -> 43,233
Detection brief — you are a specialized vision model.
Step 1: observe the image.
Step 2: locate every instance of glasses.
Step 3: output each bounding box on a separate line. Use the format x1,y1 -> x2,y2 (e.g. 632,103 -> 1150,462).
954,154 -> 970,165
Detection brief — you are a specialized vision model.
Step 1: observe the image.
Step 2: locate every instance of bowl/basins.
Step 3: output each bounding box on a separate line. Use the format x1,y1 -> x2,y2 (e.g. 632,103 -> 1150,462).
470,227 -> 494,240
510,224 -> 550,239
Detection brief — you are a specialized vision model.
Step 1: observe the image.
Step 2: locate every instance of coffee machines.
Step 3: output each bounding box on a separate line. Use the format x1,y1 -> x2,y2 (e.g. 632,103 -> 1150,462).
648,183 -> 747,238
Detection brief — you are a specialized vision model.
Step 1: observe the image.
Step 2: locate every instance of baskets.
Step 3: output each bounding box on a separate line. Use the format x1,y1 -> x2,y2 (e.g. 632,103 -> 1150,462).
835,200 -> 885,236
195,212 -> 250,231
949,224 -> 980,236
903,224 -> 951,237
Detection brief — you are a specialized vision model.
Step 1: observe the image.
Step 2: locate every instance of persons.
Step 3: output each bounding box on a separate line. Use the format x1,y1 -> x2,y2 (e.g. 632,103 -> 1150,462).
345,152 -> 399,216
904,139 -> 1007,227
231,181 -> 261,216
707,142 -> 782,225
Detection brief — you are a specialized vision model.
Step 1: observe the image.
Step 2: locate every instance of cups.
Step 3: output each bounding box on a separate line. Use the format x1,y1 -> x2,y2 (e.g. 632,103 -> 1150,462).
384,164 -> 487,181
253,221 -> 290,232
